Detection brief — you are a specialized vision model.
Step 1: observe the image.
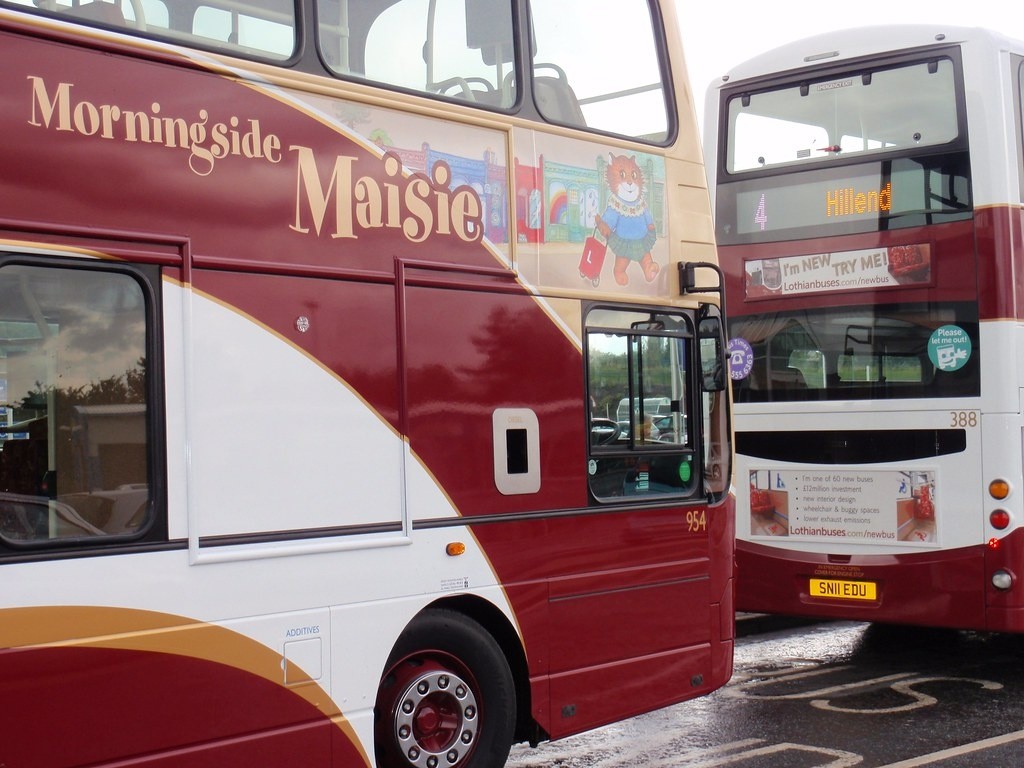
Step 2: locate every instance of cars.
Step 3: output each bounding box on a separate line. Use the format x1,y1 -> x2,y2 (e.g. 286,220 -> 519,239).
591,412 -> 688,445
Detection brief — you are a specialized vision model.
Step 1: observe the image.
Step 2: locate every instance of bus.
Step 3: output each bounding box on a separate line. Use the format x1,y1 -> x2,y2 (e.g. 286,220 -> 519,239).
0,4 -> 736,768
706,19 -> 1024,638
